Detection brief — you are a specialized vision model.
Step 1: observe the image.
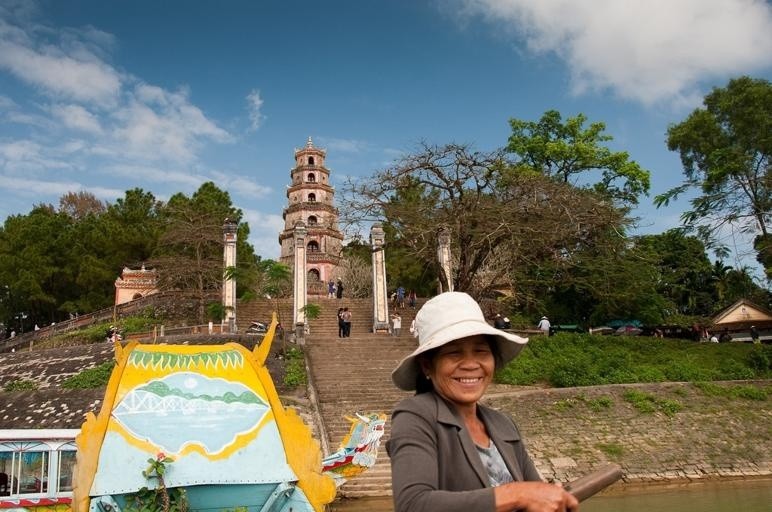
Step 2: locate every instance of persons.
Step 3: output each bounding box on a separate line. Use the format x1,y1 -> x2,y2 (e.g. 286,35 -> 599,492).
384,292 -> 580,511
107,324 -> 124,344
494,313 -> 505,329
502,316 -> 511,328
326,277 -> 418,339
750,325 -> 761,344
537,315 -> 551,335
692,322 -> 737,343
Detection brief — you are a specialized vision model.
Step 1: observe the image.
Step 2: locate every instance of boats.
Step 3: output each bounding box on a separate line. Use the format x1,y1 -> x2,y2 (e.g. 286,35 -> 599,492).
2,317 -> 393,507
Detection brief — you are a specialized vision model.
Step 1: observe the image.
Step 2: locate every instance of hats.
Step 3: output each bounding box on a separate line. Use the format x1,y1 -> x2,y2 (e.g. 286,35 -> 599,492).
495,313 -> 501,318
390,290 -> 531,393
541,316 -> 548,320
